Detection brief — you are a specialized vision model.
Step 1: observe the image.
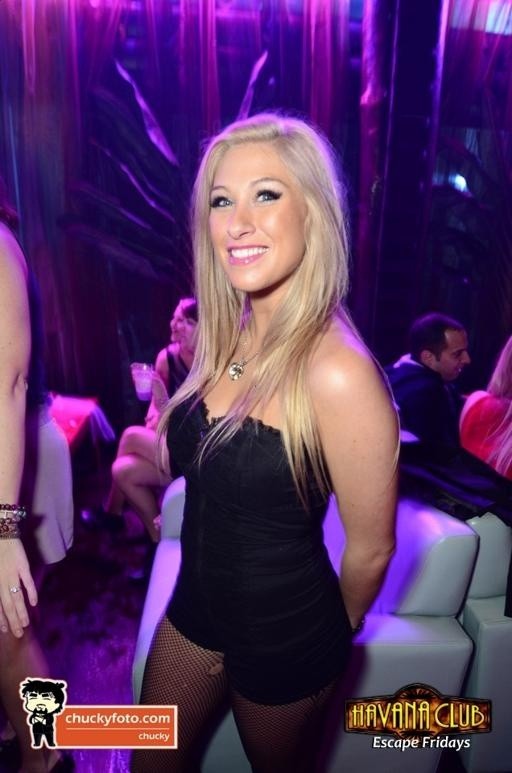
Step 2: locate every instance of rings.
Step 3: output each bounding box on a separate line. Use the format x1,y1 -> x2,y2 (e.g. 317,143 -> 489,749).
8,585 -> 22,592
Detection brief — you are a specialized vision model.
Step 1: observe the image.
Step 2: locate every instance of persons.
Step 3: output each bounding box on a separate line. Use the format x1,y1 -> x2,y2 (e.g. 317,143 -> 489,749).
458,332 -> 512,476
80,298 -> 200,586
124,110 -> 404,773
0,184 -> 83,772
384,311 -> 471,468
144,297 -> 194,429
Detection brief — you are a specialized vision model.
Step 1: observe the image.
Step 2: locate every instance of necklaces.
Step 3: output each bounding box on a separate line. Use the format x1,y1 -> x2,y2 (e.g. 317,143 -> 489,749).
226,324 -> 259,380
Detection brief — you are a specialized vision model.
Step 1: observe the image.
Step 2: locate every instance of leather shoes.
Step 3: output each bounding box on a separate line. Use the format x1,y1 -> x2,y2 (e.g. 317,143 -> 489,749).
81,506 -> 123,530
49,753 -> 75,772
128,545 -> 157,582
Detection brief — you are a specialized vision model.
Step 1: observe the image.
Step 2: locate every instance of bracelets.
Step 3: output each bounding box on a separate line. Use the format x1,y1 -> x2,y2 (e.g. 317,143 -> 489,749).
1,502 -> 29,541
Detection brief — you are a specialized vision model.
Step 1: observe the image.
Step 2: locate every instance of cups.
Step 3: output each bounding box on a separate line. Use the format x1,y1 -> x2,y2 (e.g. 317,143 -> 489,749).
130,362 -> 155,401
